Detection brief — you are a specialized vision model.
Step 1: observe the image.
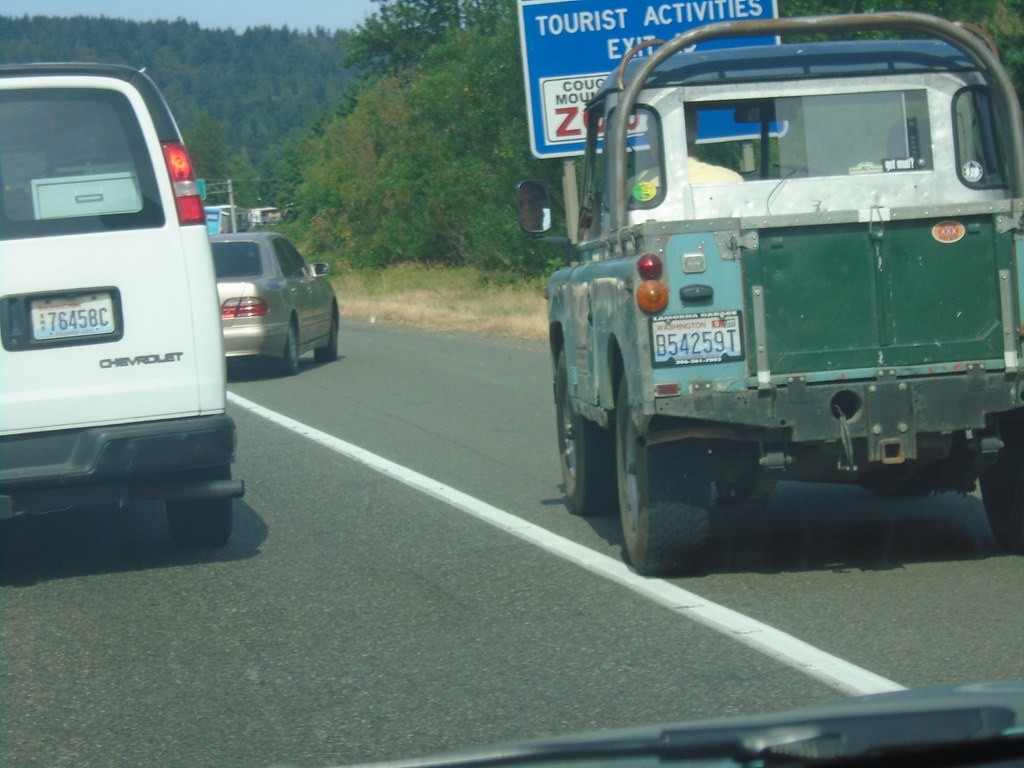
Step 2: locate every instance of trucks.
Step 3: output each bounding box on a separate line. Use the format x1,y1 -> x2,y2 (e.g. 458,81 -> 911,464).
516,11 -> 1024,576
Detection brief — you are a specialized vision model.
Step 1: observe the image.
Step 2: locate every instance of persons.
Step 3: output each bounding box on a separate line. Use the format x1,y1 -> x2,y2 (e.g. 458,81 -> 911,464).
623,105 -> 745,203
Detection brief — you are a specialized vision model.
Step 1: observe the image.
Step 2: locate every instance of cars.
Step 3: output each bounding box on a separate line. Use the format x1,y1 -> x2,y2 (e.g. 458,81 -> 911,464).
206,231 -> 340,376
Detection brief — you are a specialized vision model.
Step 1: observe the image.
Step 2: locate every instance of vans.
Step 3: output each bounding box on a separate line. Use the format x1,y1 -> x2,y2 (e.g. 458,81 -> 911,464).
0,61 -> 250,548
